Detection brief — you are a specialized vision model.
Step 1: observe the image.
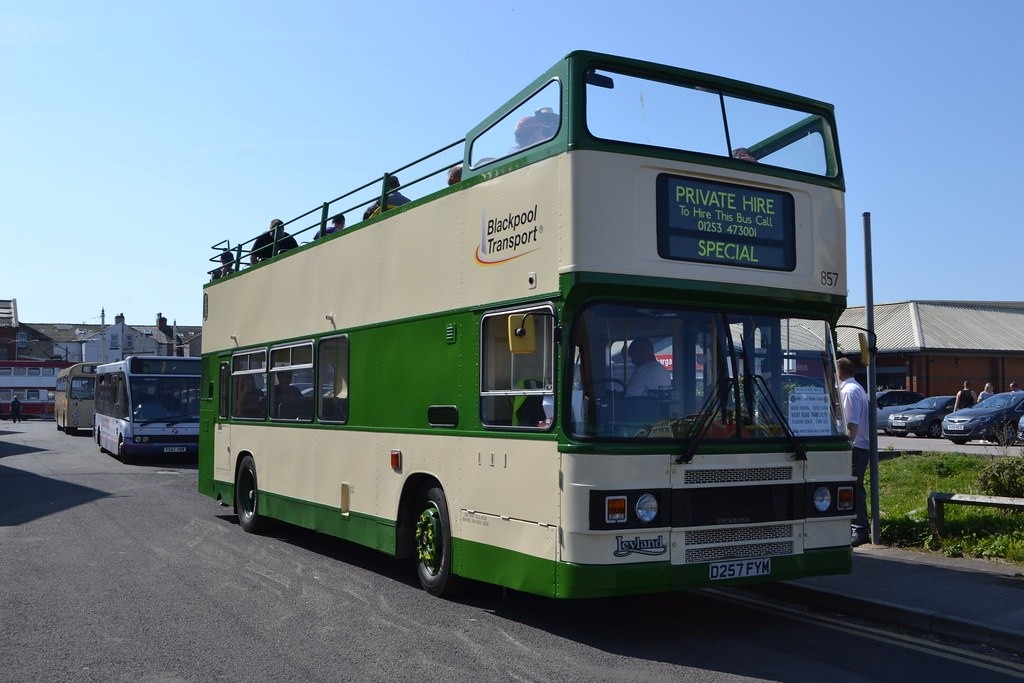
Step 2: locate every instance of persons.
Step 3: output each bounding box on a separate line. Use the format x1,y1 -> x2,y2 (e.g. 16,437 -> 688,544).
625,338 -> 672,397
250,218 -> 298,265
363,175 -> 412,221
210,248 -> 240,280
509,115 -> 550,154
835,357 -> 870,547
1009,381 -> 1023,392
977,382 -> 993,402
236,366 -> 304,417
954,380 -> 977,442
10,396 -> 23,423
313,214 -> 345,241
447,166 -> 463,185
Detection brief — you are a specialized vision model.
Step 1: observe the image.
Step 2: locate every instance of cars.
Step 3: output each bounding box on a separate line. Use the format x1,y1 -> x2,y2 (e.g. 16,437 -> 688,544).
939,390 -> 1024,447
758,373 -> 826,404
866,389 -> 927,436
887,395 -> 957,438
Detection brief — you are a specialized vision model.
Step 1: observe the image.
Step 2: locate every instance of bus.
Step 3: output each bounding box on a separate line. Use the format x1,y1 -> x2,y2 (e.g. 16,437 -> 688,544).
90,355 -> 202,465
53,360 -> 124,436
193,45 -> 877,607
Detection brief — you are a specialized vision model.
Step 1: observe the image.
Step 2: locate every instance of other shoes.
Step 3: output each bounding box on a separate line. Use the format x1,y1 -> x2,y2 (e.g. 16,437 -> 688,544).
850,531 -> 869,547
